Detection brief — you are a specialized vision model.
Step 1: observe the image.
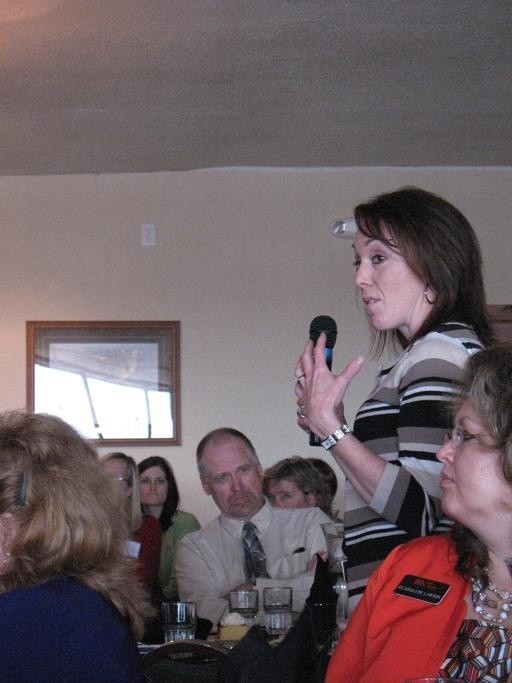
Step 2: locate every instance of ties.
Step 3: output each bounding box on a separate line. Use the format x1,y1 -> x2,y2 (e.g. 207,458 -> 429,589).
237,522 -> 273,591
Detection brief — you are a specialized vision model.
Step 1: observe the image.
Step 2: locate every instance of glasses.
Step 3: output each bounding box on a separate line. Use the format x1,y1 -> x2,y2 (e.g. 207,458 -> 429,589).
115,473 -> 130,485
442,423 -> 501,446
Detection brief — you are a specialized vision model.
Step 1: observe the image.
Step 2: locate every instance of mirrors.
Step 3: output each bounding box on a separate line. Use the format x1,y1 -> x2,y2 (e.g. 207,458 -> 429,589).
25,319 -> 181,447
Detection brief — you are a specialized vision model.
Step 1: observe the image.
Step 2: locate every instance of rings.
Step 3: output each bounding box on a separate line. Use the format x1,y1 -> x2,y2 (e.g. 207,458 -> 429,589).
296,375 -> 306,383
297,406 -> 306,418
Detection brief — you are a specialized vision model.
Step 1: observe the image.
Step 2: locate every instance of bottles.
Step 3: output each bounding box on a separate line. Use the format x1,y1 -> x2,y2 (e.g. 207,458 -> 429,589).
318,522 -> 351,655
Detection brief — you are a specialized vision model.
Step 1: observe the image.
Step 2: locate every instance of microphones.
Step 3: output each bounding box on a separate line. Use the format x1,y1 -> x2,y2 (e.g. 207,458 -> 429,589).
309,315 -> 338,447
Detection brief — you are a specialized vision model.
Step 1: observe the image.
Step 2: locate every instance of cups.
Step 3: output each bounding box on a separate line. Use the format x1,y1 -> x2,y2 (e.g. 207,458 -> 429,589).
264,586 -> 293,635
230,590 -> 258,630
161,601 -> 198,658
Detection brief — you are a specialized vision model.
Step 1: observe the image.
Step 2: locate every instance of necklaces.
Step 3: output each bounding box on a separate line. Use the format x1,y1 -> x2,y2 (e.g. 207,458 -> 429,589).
471,566 -> 512,626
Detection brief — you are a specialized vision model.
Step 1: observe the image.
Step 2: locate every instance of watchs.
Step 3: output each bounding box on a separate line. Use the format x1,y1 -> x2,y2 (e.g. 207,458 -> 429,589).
320,425 -> 353,451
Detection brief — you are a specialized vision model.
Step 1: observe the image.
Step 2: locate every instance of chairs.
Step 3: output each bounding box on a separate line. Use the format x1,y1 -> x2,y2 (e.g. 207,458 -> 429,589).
140,639 -> 231,682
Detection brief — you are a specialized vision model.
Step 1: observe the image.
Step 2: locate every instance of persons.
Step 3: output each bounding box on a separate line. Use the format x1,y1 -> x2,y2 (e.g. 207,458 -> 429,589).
0,410 -> 158,683
172,428 -> 340,626
324,345 -> 512,683
99,452 -> 201,615
262,458 -> 338,516
294,185 -> 492,620
